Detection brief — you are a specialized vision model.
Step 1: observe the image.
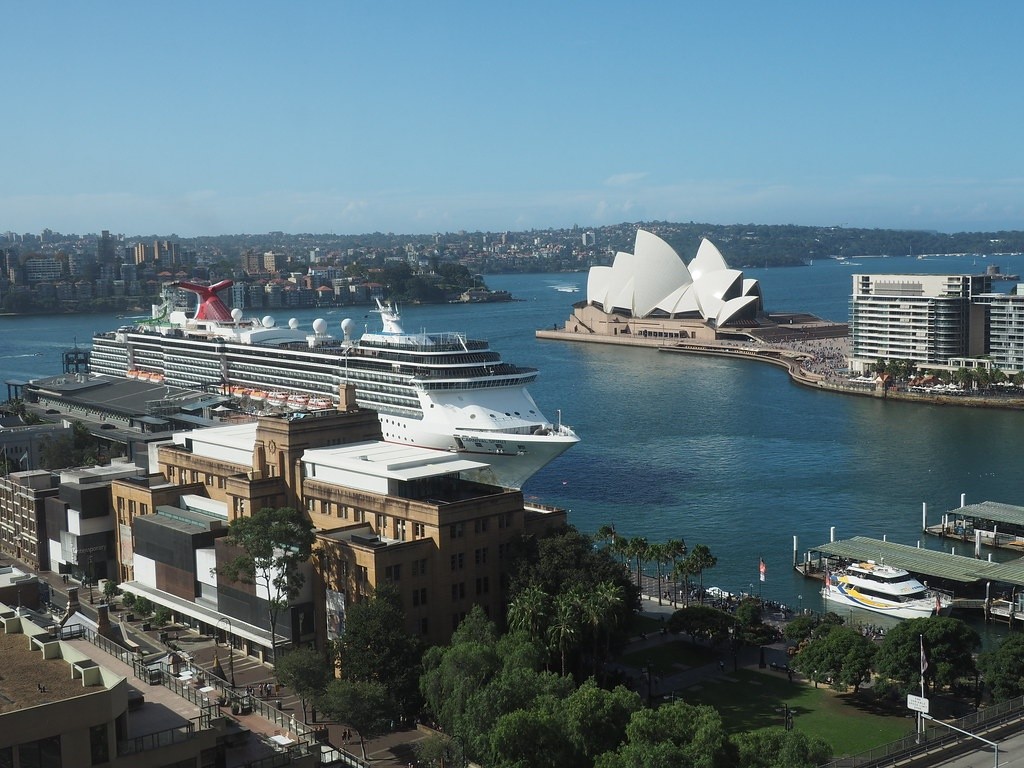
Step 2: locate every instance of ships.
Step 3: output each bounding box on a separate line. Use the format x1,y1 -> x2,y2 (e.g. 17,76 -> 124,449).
87,278 -> 581,493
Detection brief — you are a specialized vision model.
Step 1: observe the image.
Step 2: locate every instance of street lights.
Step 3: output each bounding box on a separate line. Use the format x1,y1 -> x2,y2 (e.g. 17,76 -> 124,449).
88,555 -> 95,605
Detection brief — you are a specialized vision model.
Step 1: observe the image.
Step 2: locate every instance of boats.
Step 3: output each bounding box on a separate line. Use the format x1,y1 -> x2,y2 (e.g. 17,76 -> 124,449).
821,554 -> 954,622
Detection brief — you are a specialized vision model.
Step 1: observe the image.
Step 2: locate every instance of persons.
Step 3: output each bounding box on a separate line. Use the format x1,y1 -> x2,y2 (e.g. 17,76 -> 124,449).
591,516 -> 1024,737
245,680 -> 281,702
80,579 -> 85,589
346,728 -> 353,742
62,574 -> 67,583
370,698 -> 463,768
716,313 -> 1024,407
341,728 -> 348,745
